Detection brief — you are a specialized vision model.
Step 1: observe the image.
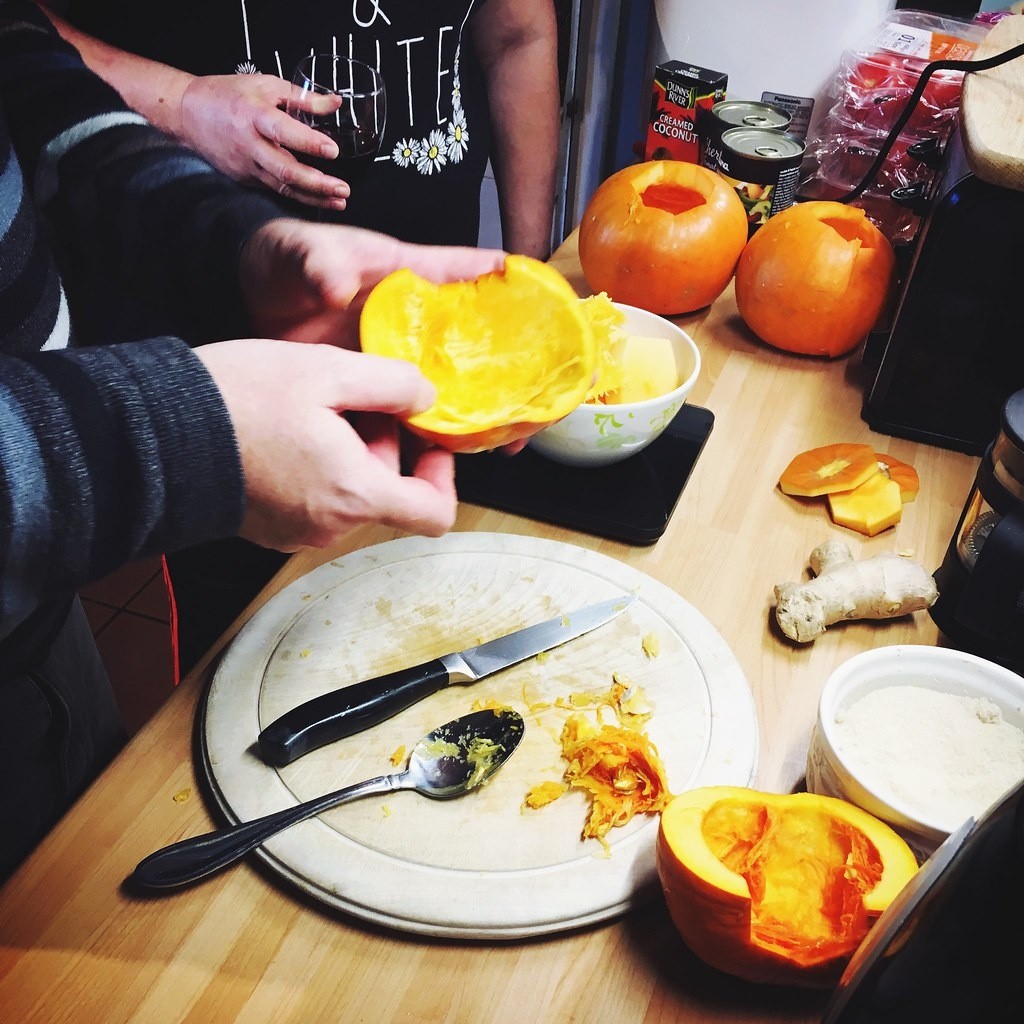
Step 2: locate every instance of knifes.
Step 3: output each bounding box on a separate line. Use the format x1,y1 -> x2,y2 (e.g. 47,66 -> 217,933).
255,589 -> 639,766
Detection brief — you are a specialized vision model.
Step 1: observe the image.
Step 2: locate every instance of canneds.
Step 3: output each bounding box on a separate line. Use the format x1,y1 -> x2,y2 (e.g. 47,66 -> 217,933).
706,103 -> 807,233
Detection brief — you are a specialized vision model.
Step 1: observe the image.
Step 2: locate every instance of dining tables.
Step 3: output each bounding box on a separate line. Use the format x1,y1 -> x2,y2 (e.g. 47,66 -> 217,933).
2,218 -> 985,1020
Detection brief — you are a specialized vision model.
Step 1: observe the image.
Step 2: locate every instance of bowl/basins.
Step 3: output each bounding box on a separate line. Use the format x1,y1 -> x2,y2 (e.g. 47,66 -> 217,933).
805,644 -> 1024,868
524,296 -> 702,463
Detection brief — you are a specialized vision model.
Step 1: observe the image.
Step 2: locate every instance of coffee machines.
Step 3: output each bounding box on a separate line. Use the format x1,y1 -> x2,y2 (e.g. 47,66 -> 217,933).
860,109 -> 1024,457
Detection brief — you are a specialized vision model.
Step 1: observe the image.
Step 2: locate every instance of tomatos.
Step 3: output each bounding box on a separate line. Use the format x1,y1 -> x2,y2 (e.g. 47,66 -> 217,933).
819,50 -> 965,195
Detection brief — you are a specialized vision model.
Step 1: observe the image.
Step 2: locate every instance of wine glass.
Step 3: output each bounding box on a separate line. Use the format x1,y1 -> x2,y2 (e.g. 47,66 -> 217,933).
284,51 -> 387,224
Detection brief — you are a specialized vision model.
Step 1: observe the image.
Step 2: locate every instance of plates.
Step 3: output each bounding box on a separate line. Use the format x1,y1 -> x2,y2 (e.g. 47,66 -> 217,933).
199,528 -> 762,940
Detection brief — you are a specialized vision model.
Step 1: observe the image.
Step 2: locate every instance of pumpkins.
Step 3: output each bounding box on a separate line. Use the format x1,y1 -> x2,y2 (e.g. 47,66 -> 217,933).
777,443 -> 920,532
358,260 -> 673,454
578,158 -> 919,358
561,723 -> 918,989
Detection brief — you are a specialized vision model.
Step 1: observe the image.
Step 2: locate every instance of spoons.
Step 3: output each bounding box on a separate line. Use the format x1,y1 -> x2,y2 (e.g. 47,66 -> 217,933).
134,707 -> 523,892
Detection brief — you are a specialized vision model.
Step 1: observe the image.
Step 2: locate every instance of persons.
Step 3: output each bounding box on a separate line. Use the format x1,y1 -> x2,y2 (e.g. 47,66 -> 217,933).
34,1 -> 566,263
1,0 -> 513,903
